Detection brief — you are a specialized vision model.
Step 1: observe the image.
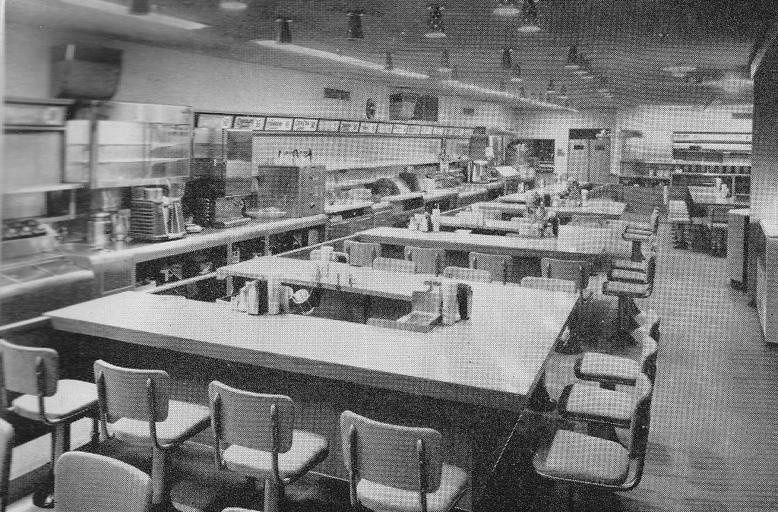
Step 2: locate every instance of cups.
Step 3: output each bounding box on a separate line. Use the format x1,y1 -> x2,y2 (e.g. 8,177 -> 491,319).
407,204 -> 443,235
307,244 -> 335,268
517,205 -> 559,238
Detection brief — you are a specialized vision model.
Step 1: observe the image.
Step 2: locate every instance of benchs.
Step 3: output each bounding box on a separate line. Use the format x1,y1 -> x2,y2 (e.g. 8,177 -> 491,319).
667,199 -> 690,249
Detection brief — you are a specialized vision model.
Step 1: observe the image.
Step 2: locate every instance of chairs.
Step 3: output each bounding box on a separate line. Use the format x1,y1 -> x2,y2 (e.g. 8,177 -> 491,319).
528,307 -> 662,512
338,411 -> 468,512
0,340 -> 98,465
207,378 -> 328,512
336,187 -> 660,345
92,356 -> 210,503
48,448 -> 156,511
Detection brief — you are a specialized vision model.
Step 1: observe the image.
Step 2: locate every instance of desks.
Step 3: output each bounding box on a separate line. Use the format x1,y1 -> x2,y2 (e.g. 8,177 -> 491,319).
40,254 -> 580,415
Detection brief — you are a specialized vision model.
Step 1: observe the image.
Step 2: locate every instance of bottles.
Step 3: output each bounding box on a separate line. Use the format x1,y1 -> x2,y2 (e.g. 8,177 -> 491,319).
87,208 -> 131,246
440,278 -> 472,326
232,276 -> 316,317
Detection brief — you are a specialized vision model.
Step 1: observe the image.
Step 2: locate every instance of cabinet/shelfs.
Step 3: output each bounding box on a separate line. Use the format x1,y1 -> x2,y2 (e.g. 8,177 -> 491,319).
619,156 -> 751,215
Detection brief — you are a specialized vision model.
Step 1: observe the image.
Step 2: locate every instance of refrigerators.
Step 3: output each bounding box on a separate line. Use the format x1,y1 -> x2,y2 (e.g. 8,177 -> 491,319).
566,128 -> 610,184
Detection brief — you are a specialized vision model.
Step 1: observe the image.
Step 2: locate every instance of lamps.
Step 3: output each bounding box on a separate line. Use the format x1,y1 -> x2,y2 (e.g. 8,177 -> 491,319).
273,0 -> 617,101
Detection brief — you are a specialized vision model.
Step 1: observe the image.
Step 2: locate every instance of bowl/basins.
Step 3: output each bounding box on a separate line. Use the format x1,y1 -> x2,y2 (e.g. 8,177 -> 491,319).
142,186 -> 165,201
